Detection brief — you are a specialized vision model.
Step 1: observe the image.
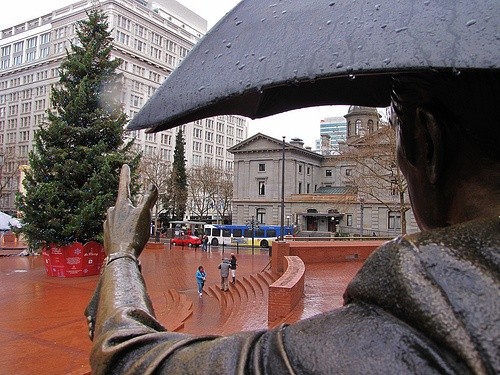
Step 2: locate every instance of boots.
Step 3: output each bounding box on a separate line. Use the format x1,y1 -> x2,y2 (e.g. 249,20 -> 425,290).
232,277 -> 235,284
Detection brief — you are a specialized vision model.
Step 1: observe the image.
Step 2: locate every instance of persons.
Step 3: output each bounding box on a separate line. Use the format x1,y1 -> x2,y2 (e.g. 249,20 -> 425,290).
373,232 -> 376,240
84,71 -> 500,375
181,229 -> 237,298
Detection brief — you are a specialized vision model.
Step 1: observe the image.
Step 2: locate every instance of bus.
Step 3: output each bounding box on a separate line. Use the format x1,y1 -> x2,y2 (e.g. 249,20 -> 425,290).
204,223 -> 294,248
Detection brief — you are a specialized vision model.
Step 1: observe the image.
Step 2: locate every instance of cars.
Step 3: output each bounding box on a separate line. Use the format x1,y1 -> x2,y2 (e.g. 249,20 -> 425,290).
170,234 -> 202,248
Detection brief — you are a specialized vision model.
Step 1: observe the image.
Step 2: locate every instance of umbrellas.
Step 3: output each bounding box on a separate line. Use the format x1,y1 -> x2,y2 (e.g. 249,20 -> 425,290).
125,1 -> 500,135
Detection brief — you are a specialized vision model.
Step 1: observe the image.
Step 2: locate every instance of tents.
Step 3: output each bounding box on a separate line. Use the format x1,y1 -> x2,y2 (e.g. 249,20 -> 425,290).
0,212 -> 27,231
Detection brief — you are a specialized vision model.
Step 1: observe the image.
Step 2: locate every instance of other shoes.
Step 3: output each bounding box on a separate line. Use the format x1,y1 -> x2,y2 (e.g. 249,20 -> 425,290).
200,293 -> 203,296
221,289 -> 229,292
198,291 -> 200,293
